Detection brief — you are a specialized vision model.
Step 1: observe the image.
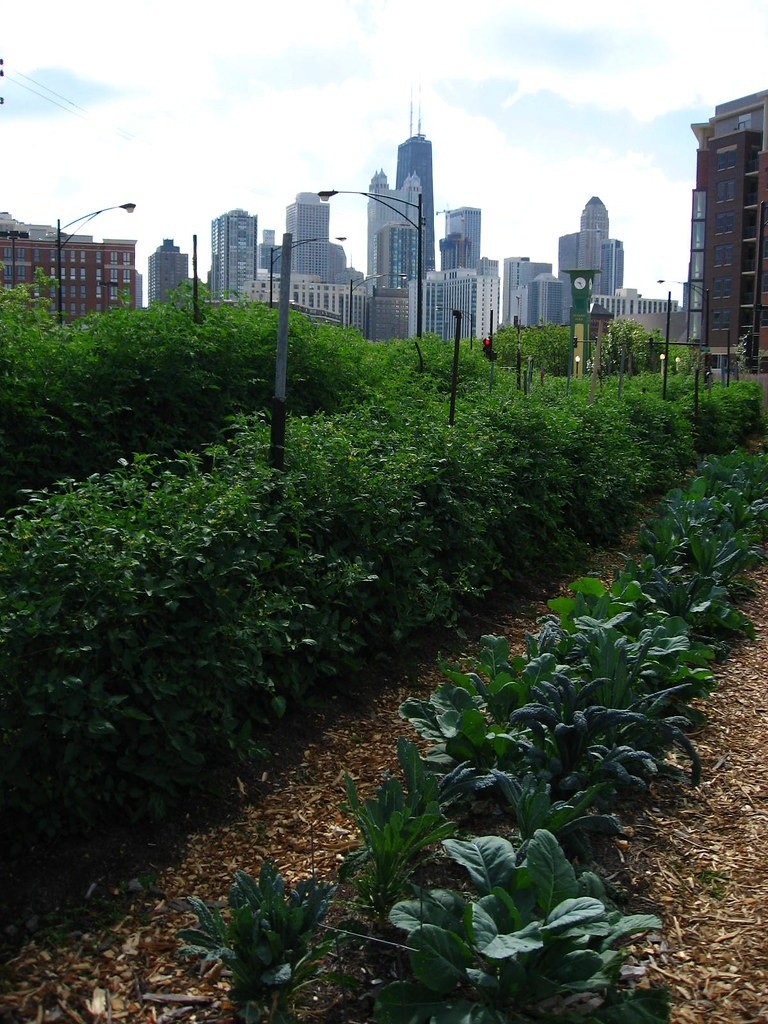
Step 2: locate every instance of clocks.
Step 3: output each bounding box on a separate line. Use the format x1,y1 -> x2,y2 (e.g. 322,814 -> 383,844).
589,278 -> 592,290
574,277 -> 586,290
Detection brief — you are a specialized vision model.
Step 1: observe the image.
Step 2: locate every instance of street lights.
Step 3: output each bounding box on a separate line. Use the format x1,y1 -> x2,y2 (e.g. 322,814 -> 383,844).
317,189 -> 426,341
657,279 -> 710,383
349,273 -> 408,326
269,235 -> 347,310
53,203 -> 138,326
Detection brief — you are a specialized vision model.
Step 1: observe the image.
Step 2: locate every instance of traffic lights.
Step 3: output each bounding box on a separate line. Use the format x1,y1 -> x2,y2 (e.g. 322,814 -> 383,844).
647,337 -> 654,349
573,337 -> 578,349
482,337 -> 492,355
